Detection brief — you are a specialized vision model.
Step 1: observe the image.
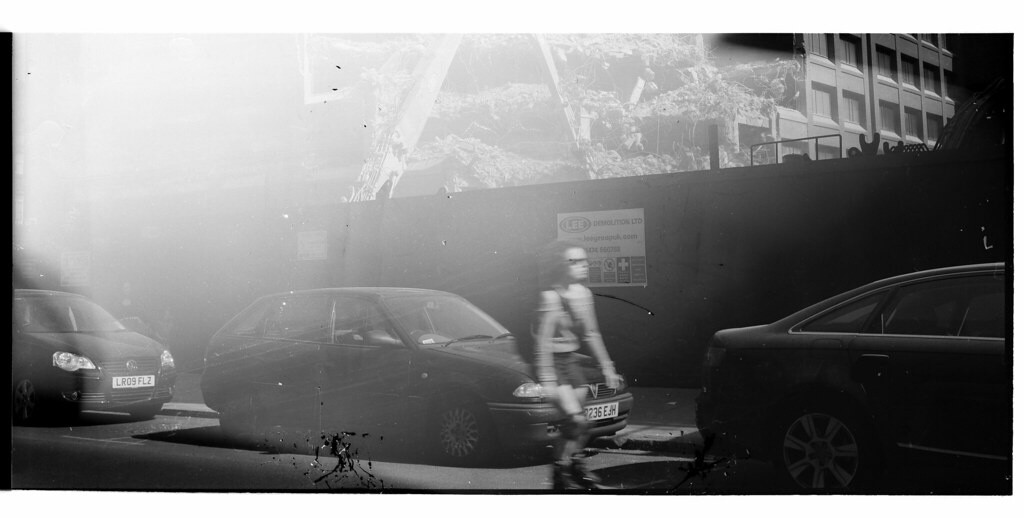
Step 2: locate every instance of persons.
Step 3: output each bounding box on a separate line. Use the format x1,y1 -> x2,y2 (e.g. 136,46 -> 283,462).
530,241 -> 625,490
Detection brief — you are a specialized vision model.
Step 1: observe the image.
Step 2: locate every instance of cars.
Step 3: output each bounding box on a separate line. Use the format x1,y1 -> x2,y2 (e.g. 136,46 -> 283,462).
697,259 -> 1008,494
12,287 -> 178,427
198,286 -> 633,467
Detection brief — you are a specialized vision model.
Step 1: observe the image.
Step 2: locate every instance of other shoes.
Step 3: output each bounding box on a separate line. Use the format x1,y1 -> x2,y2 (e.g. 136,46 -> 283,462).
571,456 -> 602,484
553,463 -> 586,489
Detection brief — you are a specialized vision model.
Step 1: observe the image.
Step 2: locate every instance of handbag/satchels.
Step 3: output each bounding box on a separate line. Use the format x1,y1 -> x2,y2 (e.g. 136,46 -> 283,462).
565,351 -> 600,388
